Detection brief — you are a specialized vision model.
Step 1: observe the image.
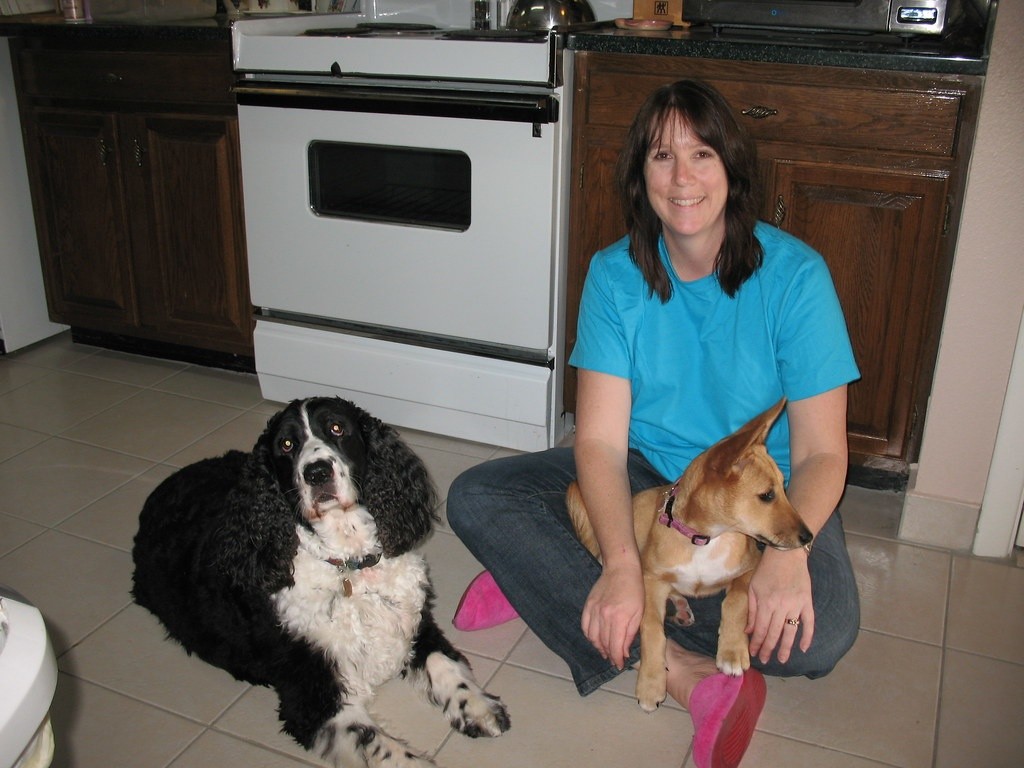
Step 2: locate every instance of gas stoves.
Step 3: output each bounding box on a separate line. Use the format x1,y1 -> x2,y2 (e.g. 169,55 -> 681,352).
231,0 -> 633,85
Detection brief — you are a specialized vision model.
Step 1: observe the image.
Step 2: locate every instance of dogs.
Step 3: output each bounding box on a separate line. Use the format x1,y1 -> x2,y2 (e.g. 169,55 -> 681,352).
564,394 -> 816,716
127,393 -> 513,768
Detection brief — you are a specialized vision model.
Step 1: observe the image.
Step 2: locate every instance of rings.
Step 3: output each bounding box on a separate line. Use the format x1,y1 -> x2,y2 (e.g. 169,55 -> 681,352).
785,618 -> 801,626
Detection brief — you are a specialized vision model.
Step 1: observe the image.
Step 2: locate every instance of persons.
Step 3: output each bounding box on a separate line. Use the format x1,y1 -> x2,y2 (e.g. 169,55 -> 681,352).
444,77 -> 861,768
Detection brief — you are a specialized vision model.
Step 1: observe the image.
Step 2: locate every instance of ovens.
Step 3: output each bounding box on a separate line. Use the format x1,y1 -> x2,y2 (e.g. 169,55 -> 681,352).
231,75 -> 572,453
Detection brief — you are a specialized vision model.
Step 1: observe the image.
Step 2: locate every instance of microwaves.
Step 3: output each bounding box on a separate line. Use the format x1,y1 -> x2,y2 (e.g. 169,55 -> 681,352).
681,0 -> 967,47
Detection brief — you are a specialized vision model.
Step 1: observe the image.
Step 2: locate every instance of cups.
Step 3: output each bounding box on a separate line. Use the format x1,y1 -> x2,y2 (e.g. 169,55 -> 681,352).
62,0 -> 85,21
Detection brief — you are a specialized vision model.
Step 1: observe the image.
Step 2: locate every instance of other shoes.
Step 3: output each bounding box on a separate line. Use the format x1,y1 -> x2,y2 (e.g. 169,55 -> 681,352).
688,667 -> 766,768
452,570 -> 521,632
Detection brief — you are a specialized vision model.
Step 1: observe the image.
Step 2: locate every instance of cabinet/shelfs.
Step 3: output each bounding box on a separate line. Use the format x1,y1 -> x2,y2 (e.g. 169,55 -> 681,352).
559,50 -> 985,491
6,36 -> 259,373
